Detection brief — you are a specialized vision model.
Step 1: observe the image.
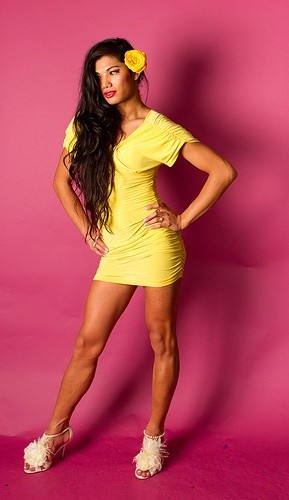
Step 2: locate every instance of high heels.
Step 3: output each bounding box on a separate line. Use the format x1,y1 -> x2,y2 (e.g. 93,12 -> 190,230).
23,426 -> 73,473
132,430 -> 169,480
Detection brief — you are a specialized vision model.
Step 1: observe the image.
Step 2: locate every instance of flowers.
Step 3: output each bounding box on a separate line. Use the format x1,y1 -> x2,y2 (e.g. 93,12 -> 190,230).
124,48 -> 146,73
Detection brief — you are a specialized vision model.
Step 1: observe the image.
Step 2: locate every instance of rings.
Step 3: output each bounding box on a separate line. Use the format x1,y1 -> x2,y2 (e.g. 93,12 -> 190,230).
92,242 -> 97,247
96,235 -> 100,240
160,217 -> 163,223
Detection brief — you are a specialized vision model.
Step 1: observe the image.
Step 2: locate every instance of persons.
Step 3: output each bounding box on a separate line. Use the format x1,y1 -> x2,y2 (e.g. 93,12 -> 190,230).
24,37 -> 237,479
